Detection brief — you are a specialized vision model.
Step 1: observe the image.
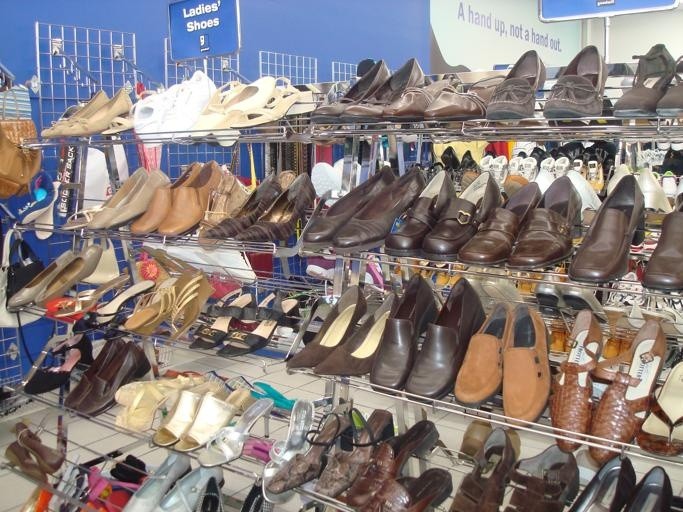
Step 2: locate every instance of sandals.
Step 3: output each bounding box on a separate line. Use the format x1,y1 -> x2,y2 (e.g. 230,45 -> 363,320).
228,76 -> 312,130
212,74 -> 276,148
192,80 -> 247,140
102,90 -> 158,136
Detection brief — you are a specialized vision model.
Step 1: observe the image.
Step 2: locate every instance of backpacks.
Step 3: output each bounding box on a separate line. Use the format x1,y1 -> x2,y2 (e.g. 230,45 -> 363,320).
0,90 -> 44,202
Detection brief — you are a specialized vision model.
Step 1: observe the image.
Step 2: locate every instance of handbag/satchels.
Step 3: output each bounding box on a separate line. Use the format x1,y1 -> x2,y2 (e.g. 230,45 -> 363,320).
0,66 -> 33,124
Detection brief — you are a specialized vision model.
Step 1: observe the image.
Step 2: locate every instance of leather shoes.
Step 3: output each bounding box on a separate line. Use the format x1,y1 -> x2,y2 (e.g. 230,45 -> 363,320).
40,88 -> 106,138
0,138 -> 683,512
654,54 -> 683,116
613,43 -> 675,117
311,59 -> 392,124
339,57 -> 425,122
420,74 -> 507,120
485,49 -> 546,120
543,41 -> 608,120
382,65 -> 463,122
62,88 -> 131,135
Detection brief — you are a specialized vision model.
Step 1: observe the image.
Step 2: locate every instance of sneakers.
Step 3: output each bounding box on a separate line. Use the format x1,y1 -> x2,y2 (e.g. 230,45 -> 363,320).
160,70 -> 217,144
130,81 -> 183,142
